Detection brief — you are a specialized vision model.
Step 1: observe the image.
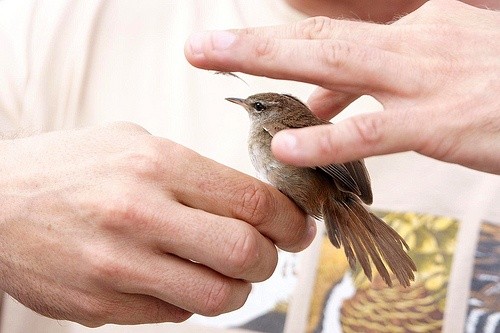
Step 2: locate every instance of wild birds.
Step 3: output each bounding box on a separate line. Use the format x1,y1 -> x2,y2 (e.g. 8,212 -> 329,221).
224,93 -> 417,288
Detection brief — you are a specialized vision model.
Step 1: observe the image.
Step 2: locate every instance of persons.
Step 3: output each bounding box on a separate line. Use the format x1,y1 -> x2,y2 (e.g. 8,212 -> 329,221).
0,0 -> 500,333
